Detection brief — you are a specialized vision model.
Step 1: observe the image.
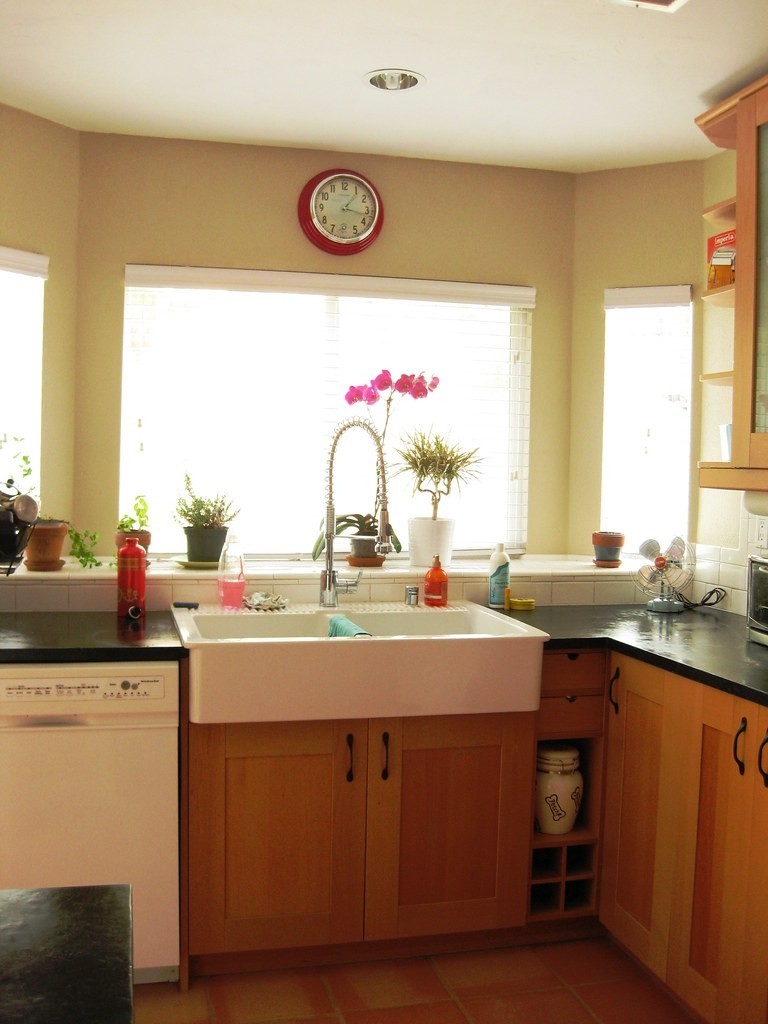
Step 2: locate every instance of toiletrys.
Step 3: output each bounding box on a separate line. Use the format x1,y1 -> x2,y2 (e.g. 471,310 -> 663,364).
217,534 -> 247,610
424,555 -> 449,607
505,588 -> 512,611
488,543 -> 511,609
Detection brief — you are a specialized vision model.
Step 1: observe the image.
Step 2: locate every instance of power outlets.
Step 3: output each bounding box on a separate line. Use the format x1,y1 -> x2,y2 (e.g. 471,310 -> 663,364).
756,518 -> 768,549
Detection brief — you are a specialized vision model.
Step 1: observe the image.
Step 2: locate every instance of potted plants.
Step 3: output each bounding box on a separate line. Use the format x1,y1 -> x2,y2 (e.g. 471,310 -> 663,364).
394,430 -> 483,567
176,476 -> 240,561
15,437 -> 102,568
115,496 -> 151,561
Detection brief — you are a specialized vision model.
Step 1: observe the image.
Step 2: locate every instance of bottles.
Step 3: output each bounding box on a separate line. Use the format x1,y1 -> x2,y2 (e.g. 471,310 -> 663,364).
489,543 -> 510,608
218,534 -> 245,611
116,538 -> 145,643
537,747 -> 581,835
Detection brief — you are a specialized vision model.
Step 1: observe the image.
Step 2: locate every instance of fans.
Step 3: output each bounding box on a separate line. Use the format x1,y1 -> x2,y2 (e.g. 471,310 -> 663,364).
629,531 -> 696,613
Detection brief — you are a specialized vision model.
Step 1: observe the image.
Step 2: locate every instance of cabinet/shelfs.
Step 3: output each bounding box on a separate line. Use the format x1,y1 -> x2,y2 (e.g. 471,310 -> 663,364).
182,649 -> 768,1023
693,197 -> 738,488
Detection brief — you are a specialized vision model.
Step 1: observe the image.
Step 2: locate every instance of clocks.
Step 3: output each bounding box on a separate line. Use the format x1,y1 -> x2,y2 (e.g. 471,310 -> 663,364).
298,169 -> 384,255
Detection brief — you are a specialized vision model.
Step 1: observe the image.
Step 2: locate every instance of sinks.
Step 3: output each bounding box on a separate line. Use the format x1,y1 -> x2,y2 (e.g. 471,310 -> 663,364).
170,601 -> 366,724
334,602 -> 551,719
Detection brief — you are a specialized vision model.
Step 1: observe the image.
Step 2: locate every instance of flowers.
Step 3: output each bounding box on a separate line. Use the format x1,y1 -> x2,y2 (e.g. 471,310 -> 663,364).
311,372 -> 439,553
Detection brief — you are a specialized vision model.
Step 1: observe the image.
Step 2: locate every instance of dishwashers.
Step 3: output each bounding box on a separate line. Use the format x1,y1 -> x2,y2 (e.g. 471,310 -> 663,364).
1,662 -> 184,985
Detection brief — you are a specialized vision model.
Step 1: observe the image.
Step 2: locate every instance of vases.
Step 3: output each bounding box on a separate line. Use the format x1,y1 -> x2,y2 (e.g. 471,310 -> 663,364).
348,539 -> 376,558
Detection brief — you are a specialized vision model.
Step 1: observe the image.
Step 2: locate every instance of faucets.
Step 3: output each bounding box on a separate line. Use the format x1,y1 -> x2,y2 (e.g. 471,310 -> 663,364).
318,415 -> 395,608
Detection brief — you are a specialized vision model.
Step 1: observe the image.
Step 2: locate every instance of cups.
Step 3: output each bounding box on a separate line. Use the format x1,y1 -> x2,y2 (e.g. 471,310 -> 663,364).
592,531 -> 624,567
